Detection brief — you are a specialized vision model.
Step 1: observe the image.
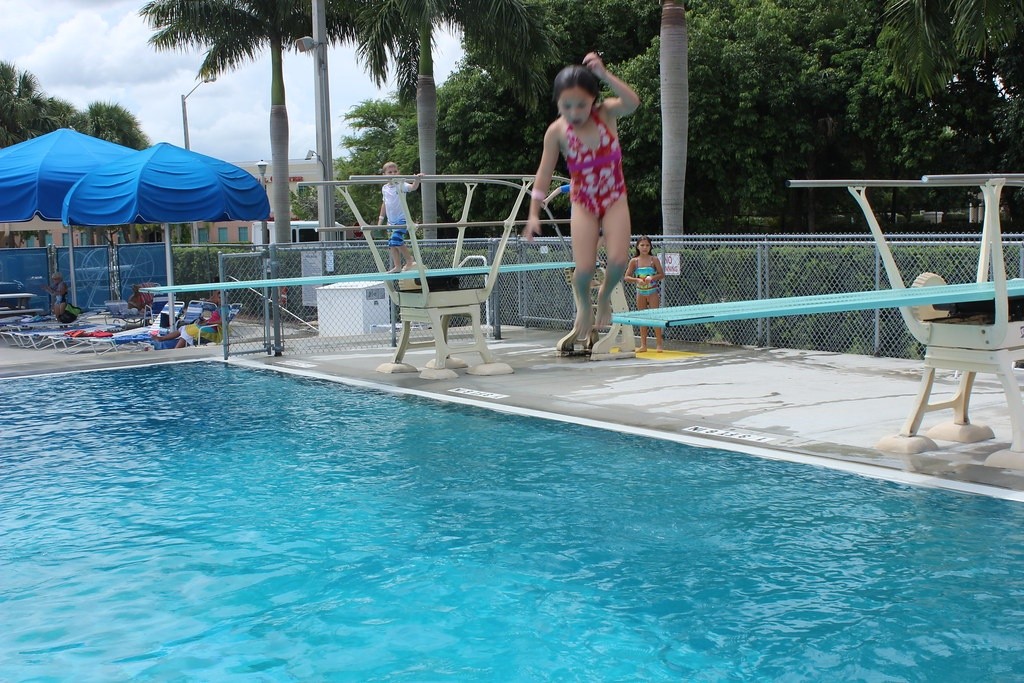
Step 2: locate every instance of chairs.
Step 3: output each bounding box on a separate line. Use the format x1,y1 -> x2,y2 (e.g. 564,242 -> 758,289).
89,327 -> 151,355
150,303 -> 243,350
104,299 -> 150,326
0,327 -> 69,348
143,300 -> 217,343
15,324 -> 96,352
115,302 -> 184,353
48,324 -> 122,355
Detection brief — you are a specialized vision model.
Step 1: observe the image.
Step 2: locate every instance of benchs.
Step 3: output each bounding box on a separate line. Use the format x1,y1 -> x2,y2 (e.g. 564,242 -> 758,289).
0,305 -> 25,311
0,308 -> 44,319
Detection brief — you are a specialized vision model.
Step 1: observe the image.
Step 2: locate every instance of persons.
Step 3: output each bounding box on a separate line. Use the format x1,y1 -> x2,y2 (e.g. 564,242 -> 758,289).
377,162 -> 424,273
541,185 -> 606,264
150,276 -> 244,348
129,284 -> 153,326
43,272 -> 68,328
521,51 -> 639,335
625,236 -> 665,352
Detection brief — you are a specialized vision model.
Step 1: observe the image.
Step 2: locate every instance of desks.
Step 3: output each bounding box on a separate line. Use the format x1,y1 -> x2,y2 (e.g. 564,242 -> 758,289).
0,292 -> 38,317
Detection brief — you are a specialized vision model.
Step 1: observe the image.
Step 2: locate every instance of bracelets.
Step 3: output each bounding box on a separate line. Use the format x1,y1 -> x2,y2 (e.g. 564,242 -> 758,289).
530,188 -> 545,201
378,216 -> 385,220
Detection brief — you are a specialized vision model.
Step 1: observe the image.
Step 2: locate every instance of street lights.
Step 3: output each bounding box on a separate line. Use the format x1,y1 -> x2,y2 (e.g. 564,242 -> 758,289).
180,75 -> 216,242
255,160 -> 269,244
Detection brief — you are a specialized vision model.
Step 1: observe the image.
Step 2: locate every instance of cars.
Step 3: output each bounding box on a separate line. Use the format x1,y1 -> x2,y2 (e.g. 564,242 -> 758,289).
251,221 -> 345,243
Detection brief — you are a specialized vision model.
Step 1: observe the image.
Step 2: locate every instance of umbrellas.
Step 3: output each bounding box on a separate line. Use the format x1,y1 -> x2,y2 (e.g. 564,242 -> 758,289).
0,128 -> 140,242
62,142 -> 270,306
